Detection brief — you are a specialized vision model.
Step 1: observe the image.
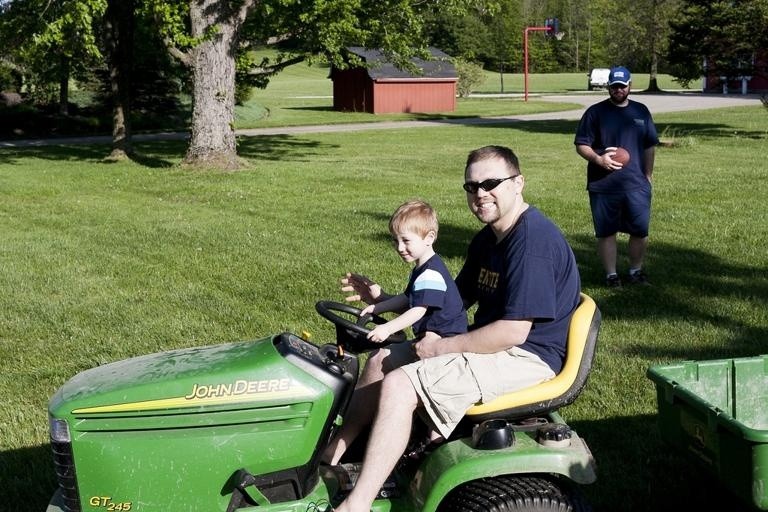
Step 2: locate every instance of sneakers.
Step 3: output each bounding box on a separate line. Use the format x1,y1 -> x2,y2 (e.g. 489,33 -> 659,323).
605,269 -> 654,289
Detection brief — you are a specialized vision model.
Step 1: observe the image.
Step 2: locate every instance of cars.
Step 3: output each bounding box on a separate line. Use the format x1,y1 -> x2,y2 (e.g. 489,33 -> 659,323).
587,68 -> 611,90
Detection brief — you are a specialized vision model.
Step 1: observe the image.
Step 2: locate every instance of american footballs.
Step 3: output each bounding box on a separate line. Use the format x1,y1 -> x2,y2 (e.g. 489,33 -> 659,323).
602,147 -> 630,167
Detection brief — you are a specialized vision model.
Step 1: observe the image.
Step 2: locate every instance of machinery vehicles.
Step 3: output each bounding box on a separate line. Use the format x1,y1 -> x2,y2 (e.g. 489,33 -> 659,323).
45,292 -> 602,512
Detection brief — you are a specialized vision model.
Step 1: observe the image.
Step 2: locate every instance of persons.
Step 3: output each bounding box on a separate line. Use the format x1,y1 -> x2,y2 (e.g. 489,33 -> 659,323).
572,63 -> 659,291
320,142 -> 582,510
314,197 -> 469,470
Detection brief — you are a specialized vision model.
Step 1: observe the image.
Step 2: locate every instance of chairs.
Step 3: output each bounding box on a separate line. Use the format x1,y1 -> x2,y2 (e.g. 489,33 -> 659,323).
462,291 -> 601,425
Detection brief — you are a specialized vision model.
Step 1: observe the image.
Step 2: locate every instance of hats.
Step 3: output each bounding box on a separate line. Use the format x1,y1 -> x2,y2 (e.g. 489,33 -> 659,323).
607,67 -> 632,88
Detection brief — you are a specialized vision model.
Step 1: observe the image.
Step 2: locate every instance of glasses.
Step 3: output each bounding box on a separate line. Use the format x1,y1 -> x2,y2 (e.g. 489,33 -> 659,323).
462,174 -> 518,194
